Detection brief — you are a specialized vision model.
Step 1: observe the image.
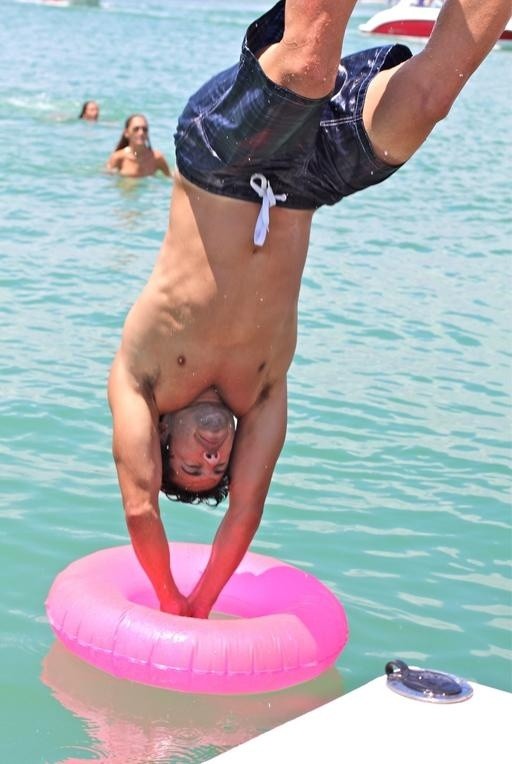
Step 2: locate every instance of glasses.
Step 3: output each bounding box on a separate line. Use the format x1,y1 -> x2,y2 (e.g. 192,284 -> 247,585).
134,127 -> 147,132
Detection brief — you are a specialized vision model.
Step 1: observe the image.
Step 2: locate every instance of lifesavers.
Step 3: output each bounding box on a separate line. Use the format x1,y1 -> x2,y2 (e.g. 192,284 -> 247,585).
45,542 -> 348,695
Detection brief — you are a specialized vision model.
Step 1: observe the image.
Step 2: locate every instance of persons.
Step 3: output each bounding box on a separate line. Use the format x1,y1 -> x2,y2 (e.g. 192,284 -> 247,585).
108,0 -> 512,619
80,101 -> 99,120
107,112 -> 172,177
